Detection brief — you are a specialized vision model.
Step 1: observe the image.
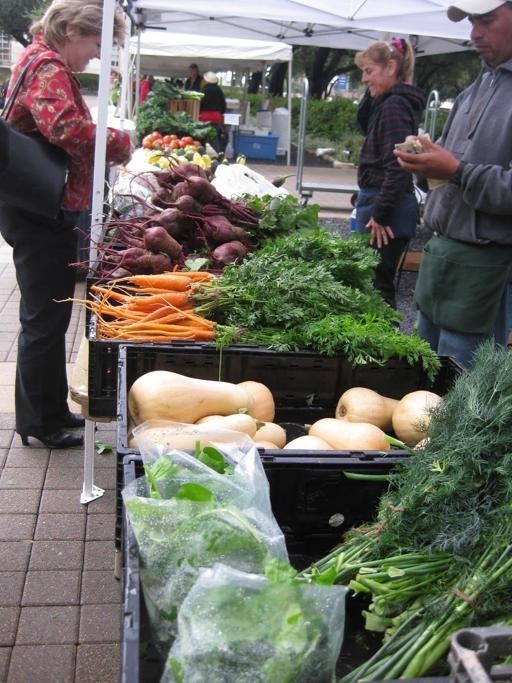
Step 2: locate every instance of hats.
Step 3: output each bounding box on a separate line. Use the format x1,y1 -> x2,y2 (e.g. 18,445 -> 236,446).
447,0 -> 512,22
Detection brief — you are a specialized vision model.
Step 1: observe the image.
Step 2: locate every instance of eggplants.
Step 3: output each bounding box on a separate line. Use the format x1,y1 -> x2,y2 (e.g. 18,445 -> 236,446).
272,174 -> 296,188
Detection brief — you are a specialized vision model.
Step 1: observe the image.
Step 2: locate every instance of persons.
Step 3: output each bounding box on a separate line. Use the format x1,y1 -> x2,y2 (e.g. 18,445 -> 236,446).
392,0 -> 511,383
131,72 -> 154,111
353,36 -> 421,326
0,0 -> 135,451
198,71 -> 227,151
183,64 -> 206,92
348,195 -> 358,232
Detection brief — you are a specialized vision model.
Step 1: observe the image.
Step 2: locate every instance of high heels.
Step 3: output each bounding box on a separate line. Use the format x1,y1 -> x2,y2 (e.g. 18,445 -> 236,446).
17,410 -> 84,448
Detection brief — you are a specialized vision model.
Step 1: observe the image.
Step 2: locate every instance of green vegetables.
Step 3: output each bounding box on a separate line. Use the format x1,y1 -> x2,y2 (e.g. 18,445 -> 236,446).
295,336 -> 512,683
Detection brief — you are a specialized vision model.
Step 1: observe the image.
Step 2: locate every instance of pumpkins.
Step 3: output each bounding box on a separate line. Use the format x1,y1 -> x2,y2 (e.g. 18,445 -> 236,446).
128,369 -> 444,452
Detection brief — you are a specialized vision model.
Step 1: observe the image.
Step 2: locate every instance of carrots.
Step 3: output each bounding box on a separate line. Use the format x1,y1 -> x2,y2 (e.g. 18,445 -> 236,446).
51,272 -> 218,342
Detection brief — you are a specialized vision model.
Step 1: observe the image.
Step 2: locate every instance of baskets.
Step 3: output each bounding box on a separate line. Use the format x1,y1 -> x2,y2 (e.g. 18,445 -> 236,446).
86,213 -> 512,683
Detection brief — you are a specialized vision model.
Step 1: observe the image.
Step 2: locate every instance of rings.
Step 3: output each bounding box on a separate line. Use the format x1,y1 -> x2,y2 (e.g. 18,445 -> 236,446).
381,231 -> 385,234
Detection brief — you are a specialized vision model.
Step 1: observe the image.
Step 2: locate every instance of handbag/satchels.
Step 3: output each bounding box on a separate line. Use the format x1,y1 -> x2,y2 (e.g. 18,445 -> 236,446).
0,115 -> 72,221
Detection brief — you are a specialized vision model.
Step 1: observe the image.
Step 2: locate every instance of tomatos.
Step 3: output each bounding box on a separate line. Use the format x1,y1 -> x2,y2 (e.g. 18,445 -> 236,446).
142,132 -> 202,149
